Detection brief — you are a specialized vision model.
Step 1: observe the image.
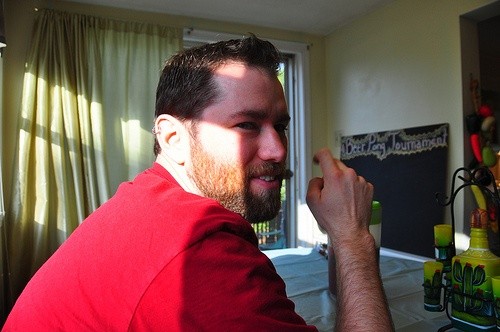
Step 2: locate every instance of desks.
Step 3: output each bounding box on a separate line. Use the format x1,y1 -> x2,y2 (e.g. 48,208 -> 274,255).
261,243 -> 461,332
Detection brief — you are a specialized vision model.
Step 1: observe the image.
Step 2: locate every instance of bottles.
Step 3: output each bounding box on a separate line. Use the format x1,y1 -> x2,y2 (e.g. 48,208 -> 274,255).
450,184 -> 500,332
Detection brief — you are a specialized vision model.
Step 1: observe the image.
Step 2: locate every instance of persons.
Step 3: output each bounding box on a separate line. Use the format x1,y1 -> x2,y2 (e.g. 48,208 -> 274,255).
2,32 -> 394,332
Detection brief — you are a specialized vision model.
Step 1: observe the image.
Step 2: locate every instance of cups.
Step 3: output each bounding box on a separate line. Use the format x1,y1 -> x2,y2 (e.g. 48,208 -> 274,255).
434,224 -> 455,270
492,276 -> 500,329
329,201 -> 382,303
424,261 -> 444,307
